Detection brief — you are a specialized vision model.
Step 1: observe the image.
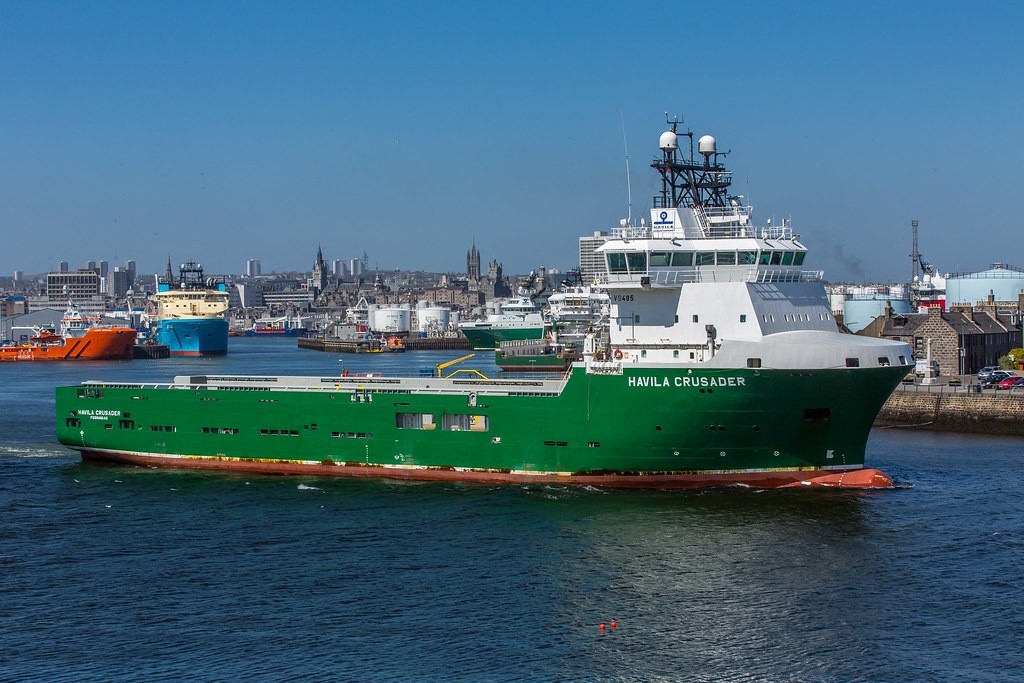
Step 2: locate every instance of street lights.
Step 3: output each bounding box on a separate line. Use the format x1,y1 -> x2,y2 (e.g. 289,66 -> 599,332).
338,359 -> 343,374
962,321 -> 975,388
956,348 -> 966,389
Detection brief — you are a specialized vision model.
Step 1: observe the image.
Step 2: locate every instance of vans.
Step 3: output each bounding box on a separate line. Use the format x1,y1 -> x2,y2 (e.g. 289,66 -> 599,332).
916,360 -> 940,377
978,365 -> 1001,380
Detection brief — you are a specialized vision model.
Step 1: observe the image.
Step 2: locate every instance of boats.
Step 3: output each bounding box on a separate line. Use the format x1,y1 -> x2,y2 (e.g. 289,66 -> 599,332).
153,257 -> 229,358
59,284 -> 102,331
53,110 -> 914,495
355,340 -> 382,353
419,367 -> 434,377
494,318 -> 612,371
227,331 -> 238,336
339,371 -> 381,377
0,321 -> 139,361
500,286 -> 611,354
457,313 -> 547,351
243,316 -> 307,337
381,337 -> 406,353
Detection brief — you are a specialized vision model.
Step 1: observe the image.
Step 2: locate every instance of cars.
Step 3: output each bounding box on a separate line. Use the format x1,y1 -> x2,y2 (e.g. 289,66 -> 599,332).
1010,378 -> 1024,390
902,371 -> 915,384
997,376 -> 1019,390
982,374 -> 1007,389
949,375 -> 962,387
990,370 -> 1019,377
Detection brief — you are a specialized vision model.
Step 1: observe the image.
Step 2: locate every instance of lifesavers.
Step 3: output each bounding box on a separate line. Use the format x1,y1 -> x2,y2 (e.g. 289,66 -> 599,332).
614,350 -> 622,360
